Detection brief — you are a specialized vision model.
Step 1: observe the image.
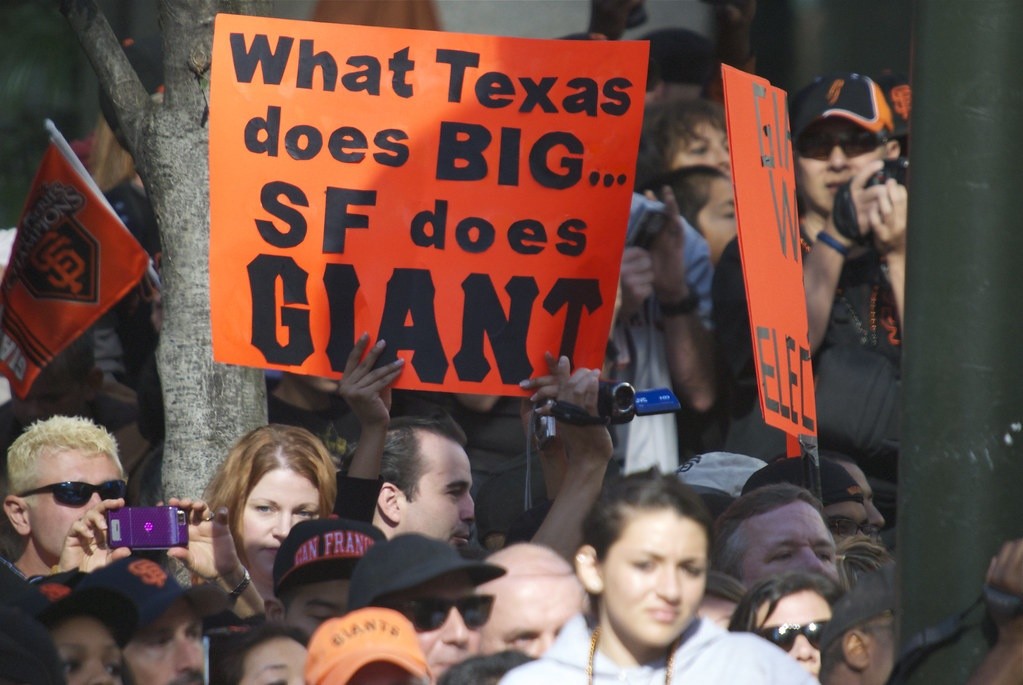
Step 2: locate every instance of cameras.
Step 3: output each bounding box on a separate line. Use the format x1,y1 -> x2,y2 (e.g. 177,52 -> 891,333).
551,379 -> 682,427
623,200 -> 671,250
834,159 -> 909,246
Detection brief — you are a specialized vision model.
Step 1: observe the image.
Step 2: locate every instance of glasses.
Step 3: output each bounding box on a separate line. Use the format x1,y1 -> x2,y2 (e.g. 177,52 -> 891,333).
385,594 -> 497,631
797,124 -> 889,158
829,519 -> 879,542
17,480 -> 127,507
755,619 -> 831,652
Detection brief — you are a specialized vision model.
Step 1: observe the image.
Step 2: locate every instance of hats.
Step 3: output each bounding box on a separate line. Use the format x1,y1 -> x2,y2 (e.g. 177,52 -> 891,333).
740,455 -> 863,509
873,69 -> 911,135
347,532 -> 508,612
305,606 -> 435,685
819,565 -> 896,650
31,567 -> 140,648
272,514 -> 389,592
791,73 -> 894,146
93,554 -> 228,630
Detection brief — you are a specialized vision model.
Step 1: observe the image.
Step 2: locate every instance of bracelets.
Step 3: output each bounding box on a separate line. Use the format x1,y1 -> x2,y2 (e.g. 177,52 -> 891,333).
227,566 -> 251,600
816,231 -> 848,255
657,282 -> 700,314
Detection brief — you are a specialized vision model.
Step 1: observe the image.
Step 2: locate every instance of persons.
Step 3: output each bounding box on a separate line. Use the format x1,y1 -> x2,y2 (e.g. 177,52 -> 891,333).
0,0 -> 1023,685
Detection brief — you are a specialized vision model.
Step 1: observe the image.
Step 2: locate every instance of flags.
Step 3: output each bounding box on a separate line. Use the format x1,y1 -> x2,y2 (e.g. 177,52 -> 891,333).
0,139 -> 150,399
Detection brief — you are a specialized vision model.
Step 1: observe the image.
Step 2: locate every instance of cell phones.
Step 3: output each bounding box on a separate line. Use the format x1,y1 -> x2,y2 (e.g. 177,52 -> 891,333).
106,505 -> 189,550
